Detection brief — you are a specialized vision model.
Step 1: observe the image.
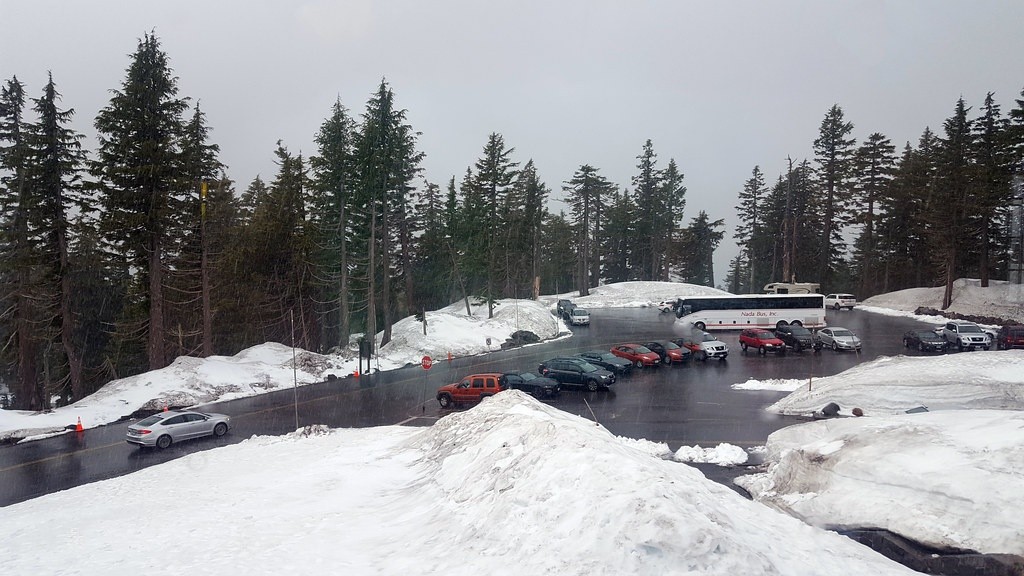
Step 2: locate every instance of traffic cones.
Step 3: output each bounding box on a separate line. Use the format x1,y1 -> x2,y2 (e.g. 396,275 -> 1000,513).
76,416 -> 83,432
163,404 -> 168,412
353,366 -> 359,376
447,351 -> 452,361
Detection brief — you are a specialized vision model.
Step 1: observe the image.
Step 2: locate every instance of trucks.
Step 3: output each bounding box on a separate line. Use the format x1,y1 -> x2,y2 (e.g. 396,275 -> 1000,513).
763,281 -> 821,294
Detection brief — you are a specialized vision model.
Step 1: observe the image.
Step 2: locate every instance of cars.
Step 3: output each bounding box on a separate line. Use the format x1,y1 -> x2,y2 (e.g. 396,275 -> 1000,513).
570,349 -> 634,373
126,409 -> 233,449
817,326 -> 863,352
933,324 -> 998,341
569,308 -> 591,326
538,355 -> 607,375
636,337 -> 706,366
557,298 -> 578,320
609,343 -> 662,369
903,329 -> 947,353
503,369 -> 562,399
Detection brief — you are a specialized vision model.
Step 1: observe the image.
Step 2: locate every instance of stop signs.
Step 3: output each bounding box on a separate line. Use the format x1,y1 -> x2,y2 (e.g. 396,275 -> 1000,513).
421,355 -> 432,370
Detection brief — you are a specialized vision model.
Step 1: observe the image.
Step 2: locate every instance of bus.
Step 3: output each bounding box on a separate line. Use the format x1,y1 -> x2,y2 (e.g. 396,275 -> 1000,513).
673,292 -> 827,335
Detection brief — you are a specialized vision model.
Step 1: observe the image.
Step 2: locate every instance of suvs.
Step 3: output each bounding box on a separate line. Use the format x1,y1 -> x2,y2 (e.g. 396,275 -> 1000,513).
996,325 -> 1024,350
436,371 -> 510,408
657,300 -> 677,313
738,327 -> 786,356
942,319 -> 993,353
825,293 -> 857,310
542,359 -> 616,392
773,325 -> 823,353
694,332 -> 730,362
